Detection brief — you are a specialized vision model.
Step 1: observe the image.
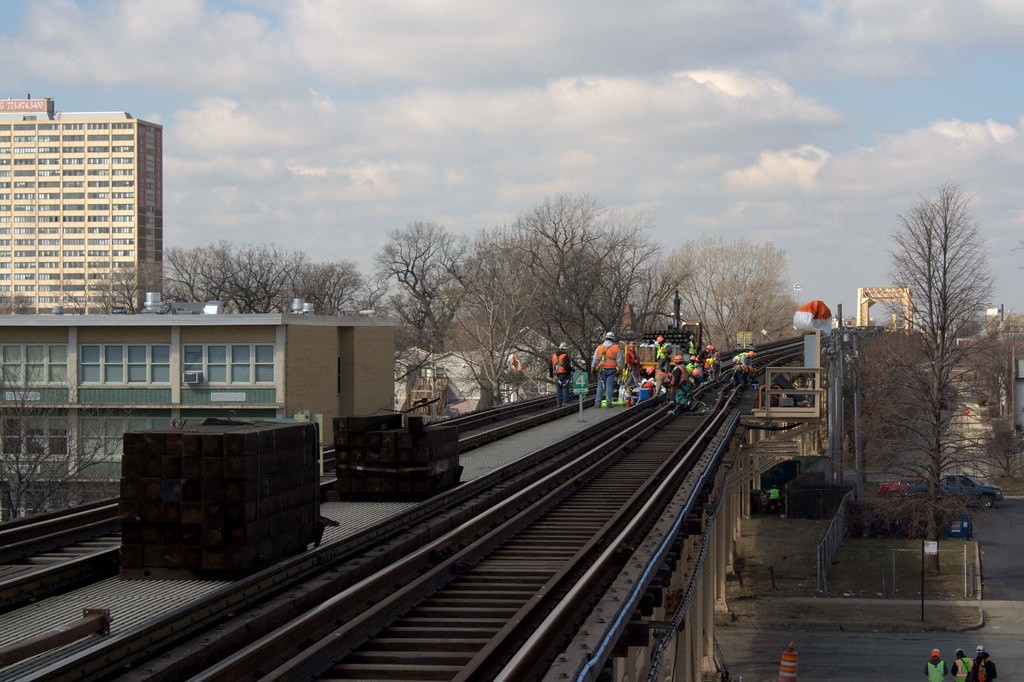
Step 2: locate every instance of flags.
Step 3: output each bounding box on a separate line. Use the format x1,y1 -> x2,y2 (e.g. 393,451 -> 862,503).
505,354 -> 525,377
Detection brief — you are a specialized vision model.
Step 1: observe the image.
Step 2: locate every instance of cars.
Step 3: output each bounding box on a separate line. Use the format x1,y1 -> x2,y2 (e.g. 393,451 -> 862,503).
877,479 -> 916,499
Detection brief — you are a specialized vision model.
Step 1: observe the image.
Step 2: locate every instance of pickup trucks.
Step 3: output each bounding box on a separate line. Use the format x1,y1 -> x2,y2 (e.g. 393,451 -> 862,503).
908,473 -> 1005,509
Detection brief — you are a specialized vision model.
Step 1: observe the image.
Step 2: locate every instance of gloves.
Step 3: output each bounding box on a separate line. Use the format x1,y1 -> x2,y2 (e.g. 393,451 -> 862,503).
591,367 -> 596,375
652,395 -> 659,400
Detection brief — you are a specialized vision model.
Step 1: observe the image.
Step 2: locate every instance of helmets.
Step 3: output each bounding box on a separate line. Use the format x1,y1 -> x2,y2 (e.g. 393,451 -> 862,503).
674,355 -> 684,362
558,342 -> 568,349
657,337 -> 663,342
748,366 -> 753,372
706,345 -> 713,351
930,649 -> 941,657
690,355 -> 696,361
692,369 -> 701,377
605,332 -> 615,339
976,645 -> 985,654
690,335 -> 694,339
954,649 -> 965,656
628,342 -> 636,346
749,351 -> 755,358
646,366 -> 655,375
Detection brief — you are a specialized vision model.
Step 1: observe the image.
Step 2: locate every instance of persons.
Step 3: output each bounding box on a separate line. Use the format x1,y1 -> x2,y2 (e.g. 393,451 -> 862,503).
951,648 -> 974,682
971,645 -> 996,682
923,648 -> 949,682
548,342 -> 575,407
625,341 -> 641,389
590,331 -> 622,408
637,334 -> 759,418
766,484 -> 781,514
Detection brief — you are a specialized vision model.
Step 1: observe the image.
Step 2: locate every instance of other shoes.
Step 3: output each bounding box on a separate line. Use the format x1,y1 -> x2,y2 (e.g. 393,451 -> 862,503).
594,404 -> 600,408
689,399 -> 699,411
562,403 -> 569,406
606,405 -> 614,408
557,405 -> 561,407
